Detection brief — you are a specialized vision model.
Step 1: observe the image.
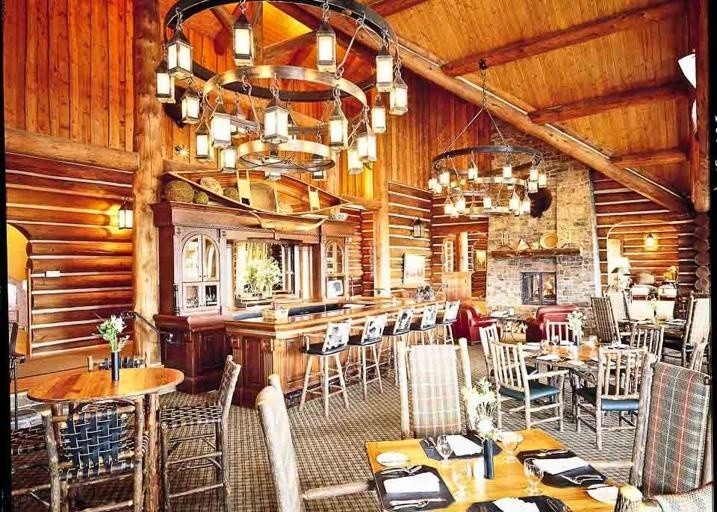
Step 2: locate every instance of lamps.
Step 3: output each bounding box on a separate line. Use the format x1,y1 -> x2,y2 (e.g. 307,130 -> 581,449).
423,60 -> 547,216
644,231 -> 657,248
675,48 -> 699,91
409,214 -> 427,241
115,191 -> 138,232
147,0 -> 415,182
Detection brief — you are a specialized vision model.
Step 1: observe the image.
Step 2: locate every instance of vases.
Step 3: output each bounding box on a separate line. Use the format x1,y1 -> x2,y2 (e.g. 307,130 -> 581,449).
112,351 -> 120,381
483,440 -> 494,480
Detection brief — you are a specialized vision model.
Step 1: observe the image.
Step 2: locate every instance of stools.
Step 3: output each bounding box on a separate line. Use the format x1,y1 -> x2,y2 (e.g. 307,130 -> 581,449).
375,307 -> 414,384
298,319 -> 349,416
347,312 -> 386,402
438,299 -> 461,357
409,303 -> 438,366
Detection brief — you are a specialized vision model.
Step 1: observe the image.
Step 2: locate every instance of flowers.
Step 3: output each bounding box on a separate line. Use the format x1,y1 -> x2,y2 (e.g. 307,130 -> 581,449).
461,374 -> 500,441
568,311 -> 587,338
242,258 -> 283,286
97,313 -> 131,351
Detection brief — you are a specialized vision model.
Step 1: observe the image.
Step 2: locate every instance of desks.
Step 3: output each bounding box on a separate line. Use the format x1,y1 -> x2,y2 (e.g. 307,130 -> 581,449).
489,310 -> 535,322
27,367 -> 183,508
364,429 -> 646,508
524,342 -> 657,422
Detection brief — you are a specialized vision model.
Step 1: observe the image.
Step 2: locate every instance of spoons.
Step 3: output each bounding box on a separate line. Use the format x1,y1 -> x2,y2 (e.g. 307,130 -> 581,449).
392,500 -> 429,511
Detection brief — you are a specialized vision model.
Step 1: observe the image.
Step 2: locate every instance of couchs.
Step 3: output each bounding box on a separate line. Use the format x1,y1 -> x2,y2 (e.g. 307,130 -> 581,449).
452,304 -> 502,346
525,304 -> 581,344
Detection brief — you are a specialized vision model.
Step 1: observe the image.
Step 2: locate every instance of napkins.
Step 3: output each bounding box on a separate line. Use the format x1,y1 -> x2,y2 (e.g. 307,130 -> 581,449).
492,496 -> 538,508
383,472 -> 440,492
447,434 -> 484,456
533,454 -> 590,475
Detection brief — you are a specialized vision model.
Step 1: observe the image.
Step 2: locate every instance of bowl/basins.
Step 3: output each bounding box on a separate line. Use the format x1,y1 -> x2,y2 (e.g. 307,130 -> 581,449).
537,230 -> 560,249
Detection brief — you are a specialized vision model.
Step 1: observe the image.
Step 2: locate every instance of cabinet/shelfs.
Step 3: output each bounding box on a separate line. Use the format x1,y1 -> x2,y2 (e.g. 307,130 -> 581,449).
157,224 -> 224,316
155,314 -> 225,394
321,235 -> 350,300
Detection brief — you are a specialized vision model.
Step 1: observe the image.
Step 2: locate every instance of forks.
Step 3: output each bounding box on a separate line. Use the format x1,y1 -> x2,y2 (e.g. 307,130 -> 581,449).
559,474 -> 603,484
380,464 -> 421,474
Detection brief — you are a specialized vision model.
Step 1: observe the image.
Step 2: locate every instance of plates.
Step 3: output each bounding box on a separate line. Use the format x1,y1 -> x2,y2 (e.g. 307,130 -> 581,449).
375,452 -> 407,466
586,484 -> 617,505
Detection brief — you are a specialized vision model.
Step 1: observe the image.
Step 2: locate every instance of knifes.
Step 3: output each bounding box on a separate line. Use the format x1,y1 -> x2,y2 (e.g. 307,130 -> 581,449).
389,498 -> 447,505
578,485 -> 613,489
546,499 -> 559,511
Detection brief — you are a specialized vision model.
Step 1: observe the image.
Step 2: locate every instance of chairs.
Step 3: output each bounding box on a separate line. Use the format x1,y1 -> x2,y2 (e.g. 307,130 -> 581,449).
590,296 -> 620,345
256,375 -> 376,508
631,325 -> 664,367
574,347 -> 650,450
583,351 -> 713,508
543,319 -> 581,346
397,337 -> 476,439
603,288 -> 633,322
41,396 -> 151,508
158,355 -> 241,508
490,341 -> 564,435
664,291 -> 712,368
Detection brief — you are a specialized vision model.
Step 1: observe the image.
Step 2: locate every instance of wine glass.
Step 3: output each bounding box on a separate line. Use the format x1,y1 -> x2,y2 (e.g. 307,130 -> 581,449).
452,462 -> 476,503
499,435 -> 524,464
523,457 -> 543,496
436,434 -> 454,464
